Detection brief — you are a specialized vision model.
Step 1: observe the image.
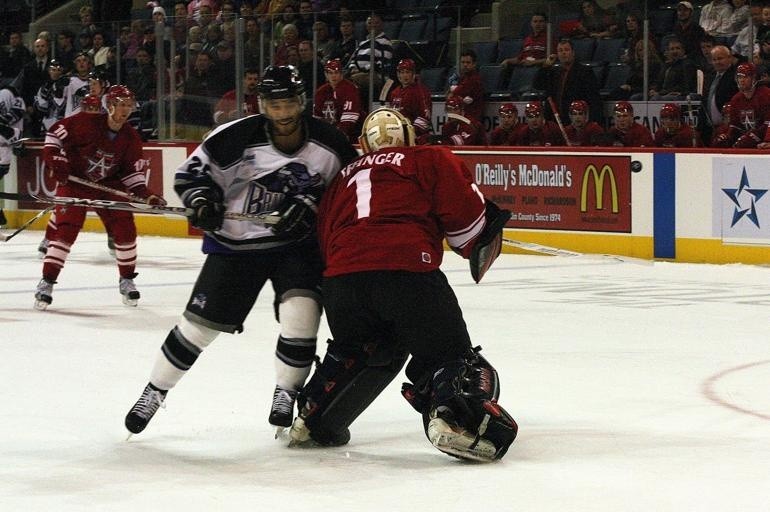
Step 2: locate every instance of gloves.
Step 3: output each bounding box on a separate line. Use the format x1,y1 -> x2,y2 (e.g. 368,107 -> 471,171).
181,184 -> 229,230
265,189 -> 320,242
51,166 -> 71,185
12,143 -> 27,157
140,188 -> 168,205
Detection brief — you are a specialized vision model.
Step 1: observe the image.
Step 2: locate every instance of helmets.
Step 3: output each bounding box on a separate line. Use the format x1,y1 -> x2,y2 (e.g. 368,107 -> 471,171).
78,94 -> 103,108
525,100 -> 544,116
736,63 -> 758,77
323,57 -> 343,71
569,99 -> 589,114
498,102 -> 519,114
660,103 -> 681,118
98,84 -> 136,109
357,107 -> 417,154
613,101 -> 634,116
396,58 -> 417,71
256,64 -> 306,101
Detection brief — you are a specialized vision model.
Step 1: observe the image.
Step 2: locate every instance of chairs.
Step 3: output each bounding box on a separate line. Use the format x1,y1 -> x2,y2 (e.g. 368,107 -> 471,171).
111,1 -> 769,102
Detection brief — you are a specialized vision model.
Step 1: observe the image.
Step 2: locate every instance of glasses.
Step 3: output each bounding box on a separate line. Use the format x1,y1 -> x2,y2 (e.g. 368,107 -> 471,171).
570,111 -> 584,115
526,114 -> 541,119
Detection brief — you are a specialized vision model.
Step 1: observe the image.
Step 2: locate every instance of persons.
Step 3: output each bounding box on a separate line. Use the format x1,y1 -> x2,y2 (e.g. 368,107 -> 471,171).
0,82 -> 28,233
32,84 -> 166,305
281,107 -> 520,464
118,63 -> 363,435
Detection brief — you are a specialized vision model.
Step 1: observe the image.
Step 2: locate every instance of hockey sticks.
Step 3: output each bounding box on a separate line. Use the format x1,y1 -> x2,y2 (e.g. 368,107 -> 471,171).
0,204 -> 55,242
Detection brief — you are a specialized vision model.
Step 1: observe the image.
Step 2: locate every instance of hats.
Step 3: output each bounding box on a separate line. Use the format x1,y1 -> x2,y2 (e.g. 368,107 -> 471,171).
675,1 -> 694,17
188,42 -> 203,52
152,6 -> 166,16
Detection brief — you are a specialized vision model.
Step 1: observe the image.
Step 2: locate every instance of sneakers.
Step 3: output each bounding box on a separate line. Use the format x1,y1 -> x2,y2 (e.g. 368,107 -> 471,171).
107,233 -> 116,250
34,278 -> 58,305
425,406 -> 497,462
124,381 -> 169,435
268,384 -> 297,428
119,274 -> 141,300
38,238 -> 49,254
289,416 -> 351,447
